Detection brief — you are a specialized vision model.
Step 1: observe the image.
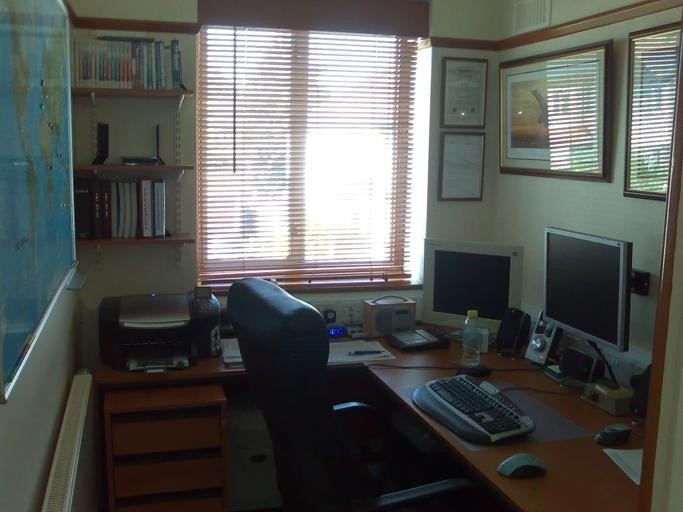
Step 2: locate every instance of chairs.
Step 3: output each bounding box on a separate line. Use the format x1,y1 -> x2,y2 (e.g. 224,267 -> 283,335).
226,275 -> 482,511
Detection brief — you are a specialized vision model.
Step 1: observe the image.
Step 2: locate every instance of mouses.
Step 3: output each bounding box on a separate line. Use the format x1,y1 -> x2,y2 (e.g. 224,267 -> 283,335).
594,421 -> 633,446
496,452 -> 548,479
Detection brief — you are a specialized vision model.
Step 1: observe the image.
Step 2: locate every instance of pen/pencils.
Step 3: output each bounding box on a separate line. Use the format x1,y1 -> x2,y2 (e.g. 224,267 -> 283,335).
348,350 -> 385,355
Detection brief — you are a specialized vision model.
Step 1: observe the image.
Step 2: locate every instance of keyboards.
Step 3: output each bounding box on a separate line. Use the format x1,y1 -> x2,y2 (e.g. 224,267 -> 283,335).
411,373 -> 536,445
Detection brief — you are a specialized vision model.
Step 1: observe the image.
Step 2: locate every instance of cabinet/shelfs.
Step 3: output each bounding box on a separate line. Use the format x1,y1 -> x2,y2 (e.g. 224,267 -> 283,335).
102,382 -> 230,512
70,86 -> 194,246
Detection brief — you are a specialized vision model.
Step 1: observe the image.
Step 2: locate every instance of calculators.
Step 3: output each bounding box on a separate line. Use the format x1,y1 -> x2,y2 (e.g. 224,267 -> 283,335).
389,327 -> 449,351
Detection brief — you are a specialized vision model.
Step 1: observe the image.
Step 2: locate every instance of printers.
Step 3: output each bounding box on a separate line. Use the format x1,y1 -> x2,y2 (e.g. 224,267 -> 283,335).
97,290 -> 219,375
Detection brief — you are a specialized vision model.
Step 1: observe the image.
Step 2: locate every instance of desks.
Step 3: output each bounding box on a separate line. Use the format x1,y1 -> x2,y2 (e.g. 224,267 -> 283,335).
93,323 -> 647,511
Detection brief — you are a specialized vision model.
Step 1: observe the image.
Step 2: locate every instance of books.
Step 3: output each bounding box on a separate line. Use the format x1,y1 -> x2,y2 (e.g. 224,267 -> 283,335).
70,30 -> 186,239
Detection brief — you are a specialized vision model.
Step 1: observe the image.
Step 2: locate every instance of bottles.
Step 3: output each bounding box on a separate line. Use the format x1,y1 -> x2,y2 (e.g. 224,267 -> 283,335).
461,310 -> 481,367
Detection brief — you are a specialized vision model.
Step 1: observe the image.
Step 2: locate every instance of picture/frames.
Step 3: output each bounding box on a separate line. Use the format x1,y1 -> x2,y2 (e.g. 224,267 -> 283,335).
436,56 -> 488,201
498,38 -> 612,185
621,21 -> 681,201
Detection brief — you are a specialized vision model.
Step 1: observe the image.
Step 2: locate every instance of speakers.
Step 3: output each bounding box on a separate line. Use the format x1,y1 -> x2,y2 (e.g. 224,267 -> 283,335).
630,363 -> 652,426
496,307 -> 530,357
362,294 -> 416,336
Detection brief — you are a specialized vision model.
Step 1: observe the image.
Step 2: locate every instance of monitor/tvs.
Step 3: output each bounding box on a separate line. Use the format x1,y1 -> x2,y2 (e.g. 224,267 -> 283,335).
423,238 -> 523,343
543,227 -> 632,353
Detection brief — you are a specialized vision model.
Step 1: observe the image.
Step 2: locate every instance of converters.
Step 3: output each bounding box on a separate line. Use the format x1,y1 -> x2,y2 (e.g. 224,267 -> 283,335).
324,310 -> 337,323
